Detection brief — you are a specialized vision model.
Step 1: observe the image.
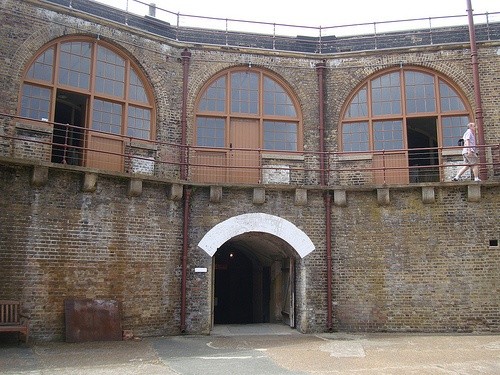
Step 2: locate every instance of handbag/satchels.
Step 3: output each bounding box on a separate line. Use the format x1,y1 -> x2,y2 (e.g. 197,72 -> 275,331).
458,139 -> 464,147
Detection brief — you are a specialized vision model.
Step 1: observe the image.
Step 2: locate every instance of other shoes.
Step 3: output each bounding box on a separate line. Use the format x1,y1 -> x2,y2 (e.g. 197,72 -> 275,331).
452,178 -> 459,181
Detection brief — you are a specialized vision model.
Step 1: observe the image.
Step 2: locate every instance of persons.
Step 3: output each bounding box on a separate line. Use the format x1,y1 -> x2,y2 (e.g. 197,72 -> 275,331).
451,122 -> 482,182
